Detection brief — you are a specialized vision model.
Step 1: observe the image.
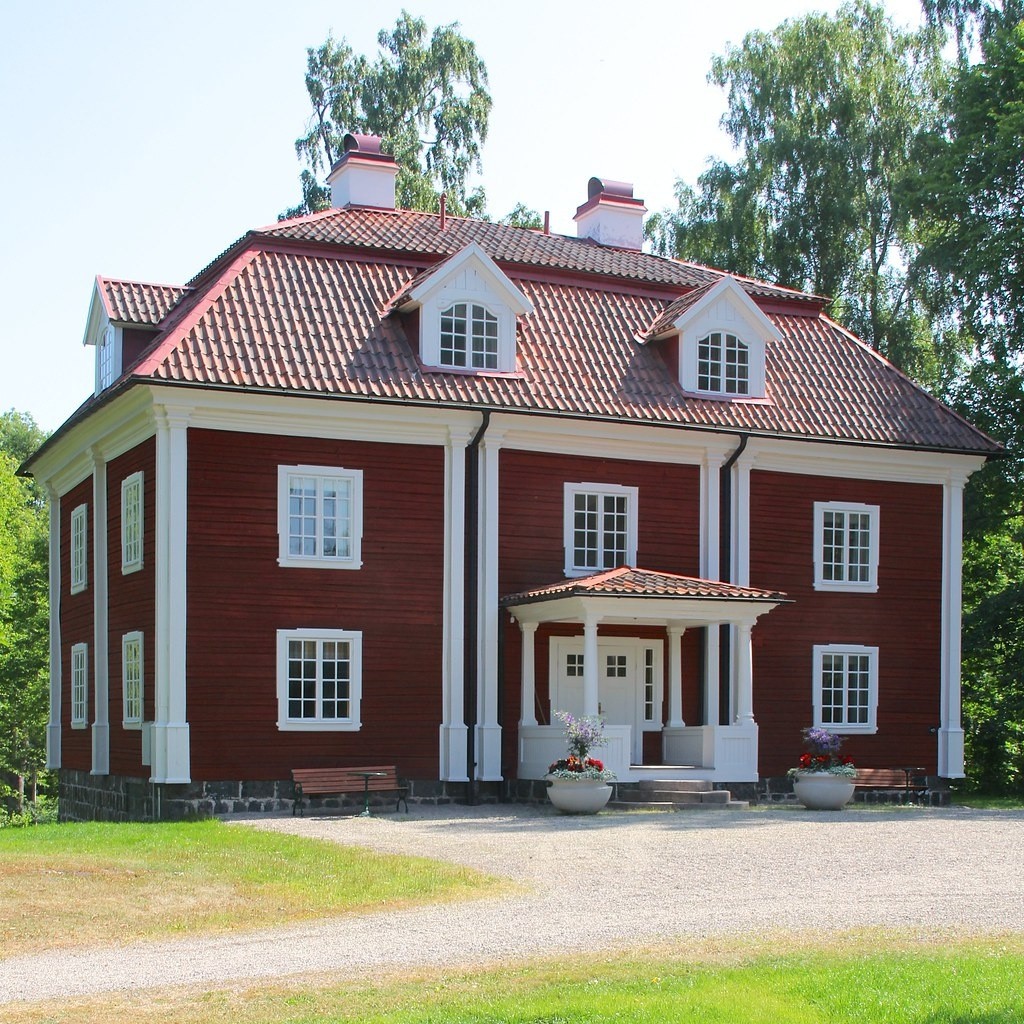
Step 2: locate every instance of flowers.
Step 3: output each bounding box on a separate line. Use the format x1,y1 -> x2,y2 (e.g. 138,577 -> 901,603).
544,708 -> 619,780
787,727 -> 857,784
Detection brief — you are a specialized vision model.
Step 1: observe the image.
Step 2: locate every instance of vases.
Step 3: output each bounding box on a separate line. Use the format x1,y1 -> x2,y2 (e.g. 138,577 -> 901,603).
793,769 -> 857,812
544,774 -> 615,815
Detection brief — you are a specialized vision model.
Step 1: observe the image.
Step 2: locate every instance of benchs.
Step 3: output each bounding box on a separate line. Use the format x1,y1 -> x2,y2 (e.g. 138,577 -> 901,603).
849,768 -> 930,806
291,764 -> 410,817
